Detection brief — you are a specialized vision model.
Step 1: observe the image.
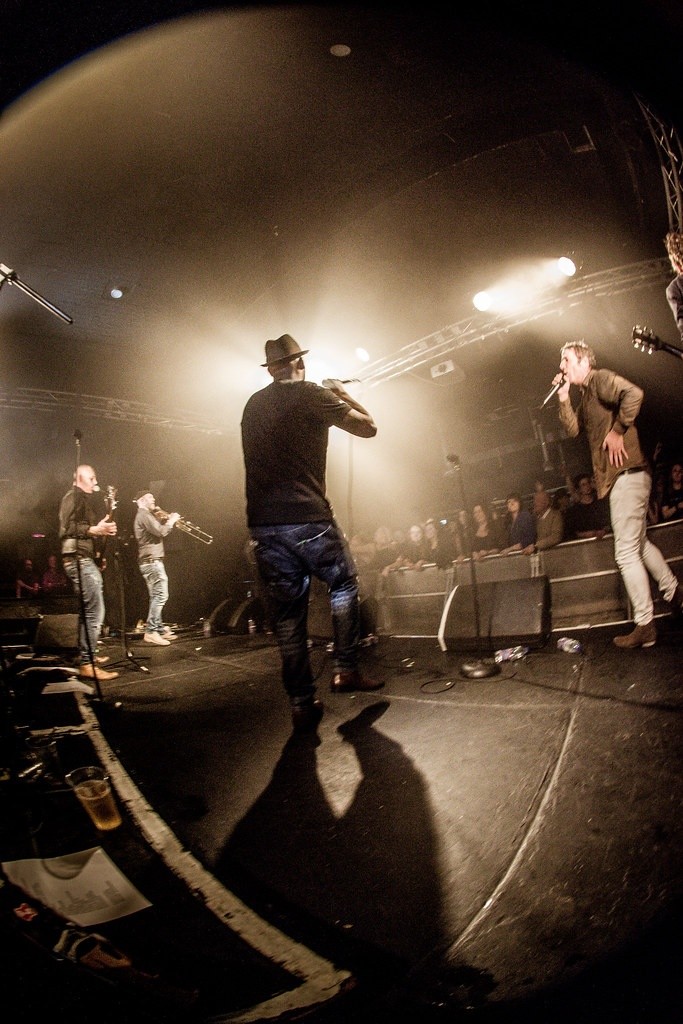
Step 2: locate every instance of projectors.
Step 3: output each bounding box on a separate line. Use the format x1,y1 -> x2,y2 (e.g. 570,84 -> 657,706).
430,360 -> 466,386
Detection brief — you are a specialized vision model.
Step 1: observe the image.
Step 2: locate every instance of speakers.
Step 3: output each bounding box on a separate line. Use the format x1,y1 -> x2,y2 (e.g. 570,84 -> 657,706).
307,594 -> 378,640
206,599 -> 240,632
226,596 -> 263,633
443,574 -> 551,652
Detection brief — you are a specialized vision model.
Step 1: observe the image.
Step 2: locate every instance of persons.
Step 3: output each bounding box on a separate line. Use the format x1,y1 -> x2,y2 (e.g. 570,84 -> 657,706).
348,442 -> 683,578
134,490 -> 182,646
15,555 -> 68,599
664,232 -> 683,342
59,464 -> 119,681
551,342 -> 683,649
240,334 -> 386,718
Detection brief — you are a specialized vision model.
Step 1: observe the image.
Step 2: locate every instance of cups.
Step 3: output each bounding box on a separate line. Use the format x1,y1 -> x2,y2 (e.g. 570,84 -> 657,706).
248,619 -> 257,634
202,620 -> 211,638
64,767 -> 122,831
508,646 -> 526,662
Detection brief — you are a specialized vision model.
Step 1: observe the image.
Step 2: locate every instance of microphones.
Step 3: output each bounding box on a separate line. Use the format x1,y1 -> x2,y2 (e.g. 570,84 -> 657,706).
93,486 -> 109,495
539,373 -> 566,410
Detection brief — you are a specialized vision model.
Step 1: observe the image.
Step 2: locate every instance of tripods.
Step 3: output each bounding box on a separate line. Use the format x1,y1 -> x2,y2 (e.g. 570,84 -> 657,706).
98,556 -> 152,674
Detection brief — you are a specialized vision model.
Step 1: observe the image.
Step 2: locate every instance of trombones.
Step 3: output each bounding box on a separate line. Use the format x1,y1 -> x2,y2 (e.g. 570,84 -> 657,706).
154,507 -> 214,545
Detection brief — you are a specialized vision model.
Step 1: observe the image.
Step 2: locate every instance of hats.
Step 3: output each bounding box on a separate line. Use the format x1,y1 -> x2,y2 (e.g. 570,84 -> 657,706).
261,334 -> 309,366
132,491 -> 150,502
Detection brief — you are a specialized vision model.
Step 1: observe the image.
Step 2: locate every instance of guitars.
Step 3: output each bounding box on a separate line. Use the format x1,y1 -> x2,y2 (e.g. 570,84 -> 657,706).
95,484 -> 118,572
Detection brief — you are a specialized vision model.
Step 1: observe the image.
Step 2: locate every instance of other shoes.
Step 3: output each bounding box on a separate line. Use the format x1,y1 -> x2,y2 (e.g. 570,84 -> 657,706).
292,697 -> 323,714
670,584 -> 683,622
144,632 -> 170,645
330,670 -> 385,693
161,634 -> 177,640
80,664 -> 119,679
93,656 -> 109,662
613,620 -> 656,647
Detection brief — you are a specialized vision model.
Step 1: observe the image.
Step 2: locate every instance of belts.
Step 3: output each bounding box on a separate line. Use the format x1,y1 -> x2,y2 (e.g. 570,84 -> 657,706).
142,559 -> 164,563
619,467 -> 642,474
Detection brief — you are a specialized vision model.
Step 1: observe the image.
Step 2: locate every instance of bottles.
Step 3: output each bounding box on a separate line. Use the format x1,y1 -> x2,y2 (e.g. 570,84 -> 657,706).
556,637 -> 584,654
494,645 -> 529,662
